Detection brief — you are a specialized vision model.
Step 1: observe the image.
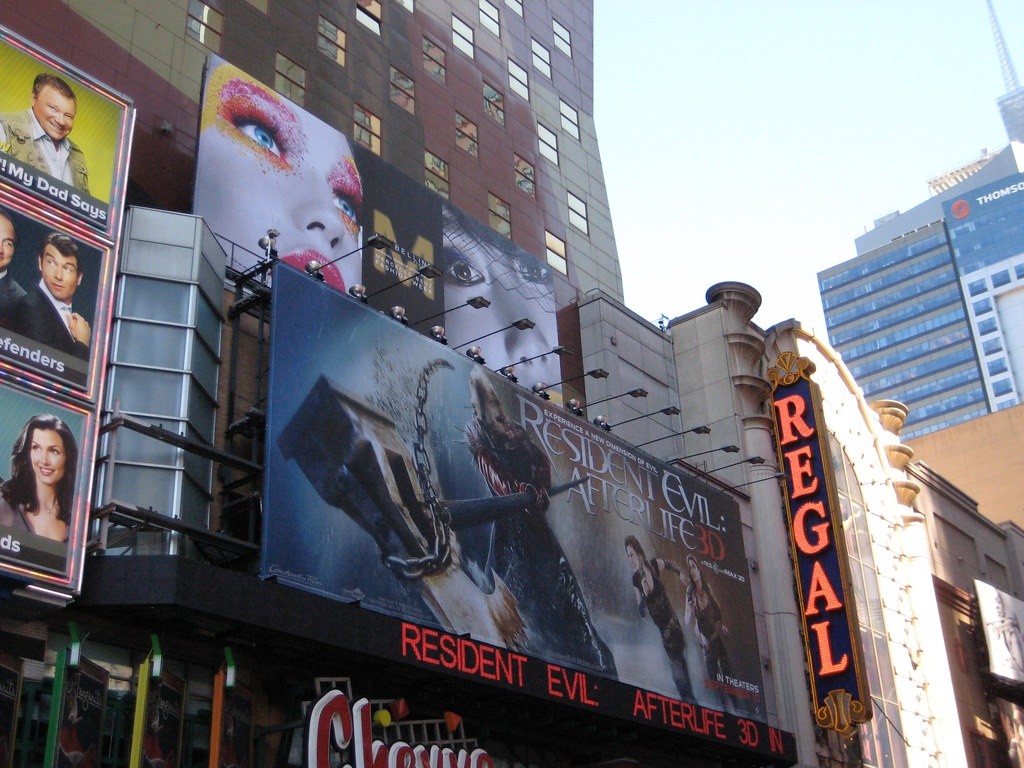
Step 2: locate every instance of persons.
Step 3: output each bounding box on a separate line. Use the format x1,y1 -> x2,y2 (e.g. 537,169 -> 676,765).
192,52 -> 364,301
443,194 -> 563,409
0,34 -> 129,545
624,534 -> 738,718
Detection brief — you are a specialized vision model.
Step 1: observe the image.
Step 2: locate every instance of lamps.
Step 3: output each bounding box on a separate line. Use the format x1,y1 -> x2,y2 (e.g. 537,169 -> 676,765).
390,305 -> 408,324
582,389 -> 649,409
260,236 -> 279,256
567,399 -> 584,416
363,265 -> 444,304
533,369 -> 610,393
609,406 -> 681,429
636,425 -> 711,448
495,345 -> 573,373
532,382 -> 549,399
722,473 -> 790,493
453,318 -> 536,350
594,415 -> 611,431
407,296 -> 492,328
430,325 -> 448,345
681,445 -> 740,460
306,260 -> 326,279
267,228 -> 281,260
695,457 -> 766,479
466,345 -> 486,364
314,234 -> 394,279
350,284 -> 367,302
501,365 -> 519,383
